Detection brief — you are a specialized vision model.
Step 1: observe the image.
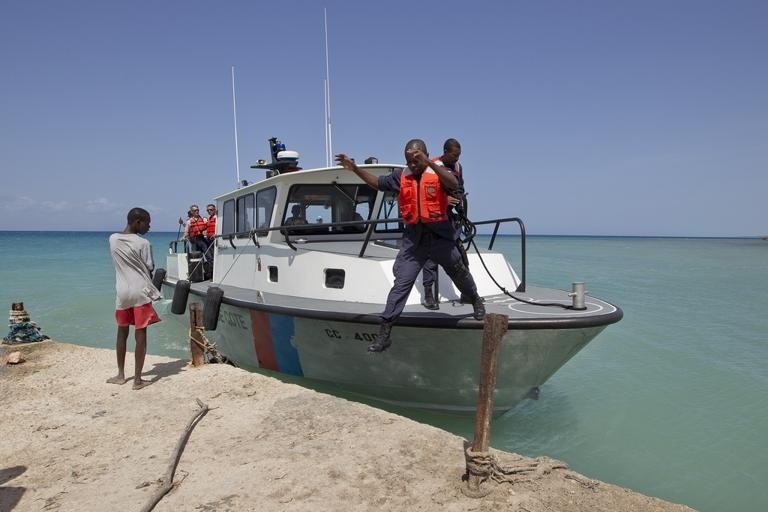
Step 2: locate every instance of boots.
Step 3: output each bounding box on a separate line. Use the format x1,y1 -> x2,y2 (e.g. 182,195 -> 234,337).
460,293 -> 486,320
423,286 -> 439,310
367,321 -> 393,353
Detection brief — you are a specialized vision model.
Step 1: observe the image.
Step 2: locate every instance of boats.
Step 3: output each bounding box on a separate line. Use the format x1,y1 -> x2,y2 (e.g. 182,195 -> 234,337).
153,5 -> 625,421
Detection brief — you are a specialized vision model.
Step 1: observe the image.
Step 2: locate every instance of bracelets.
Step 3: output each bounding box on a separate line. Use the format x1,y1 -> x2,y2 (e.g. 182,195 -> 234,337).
430,162 -> 437,169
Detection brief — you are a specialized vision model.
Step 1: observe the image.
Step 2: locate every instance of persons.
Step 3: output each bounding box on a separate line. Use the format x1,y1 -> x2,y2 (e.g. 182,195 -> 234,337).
177,210 -> 192,229
353,203 -> 365,230
315,215 -> 323,225
334,139 -> 489,355
421,138 -> 485,310
283,204 -> 308,229
203,203 -> 217,241
106,207 -> 162,391
180,204 -> 213,278
